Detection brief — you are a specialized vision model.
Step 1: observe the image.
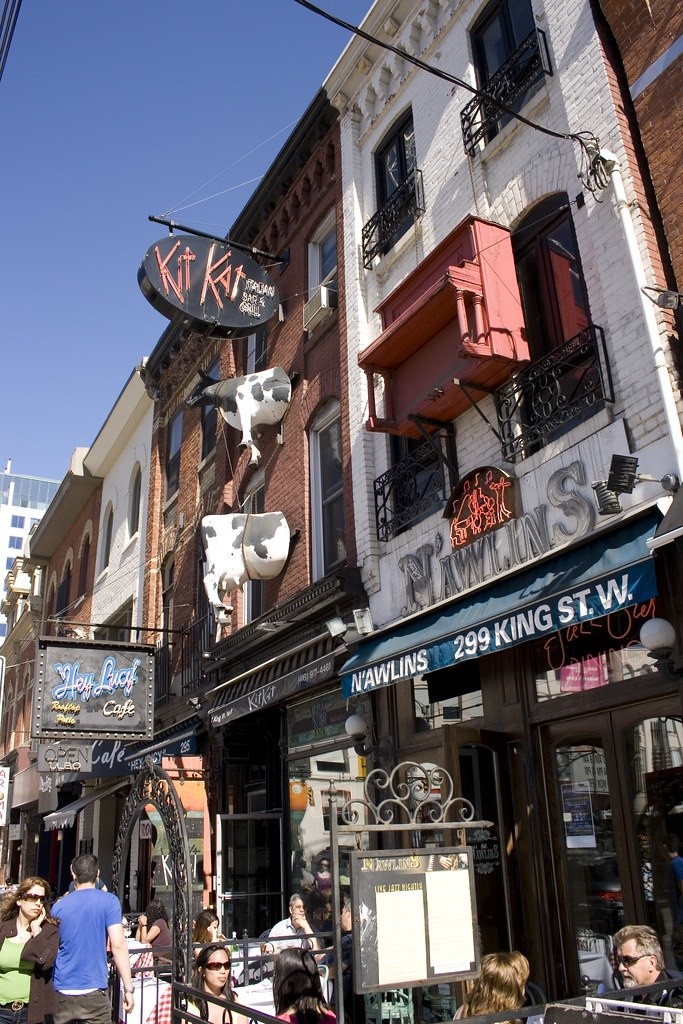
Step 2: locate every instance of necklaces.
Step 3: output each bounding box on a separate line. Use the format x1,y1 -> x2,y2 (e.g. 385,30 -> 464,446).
17,930 -> 26,938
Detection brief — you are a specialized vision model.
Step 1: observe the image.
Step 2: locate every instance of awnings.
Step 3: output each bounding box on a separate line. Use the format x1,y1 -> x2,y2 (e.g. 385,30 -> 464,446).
337,492 -> 674,700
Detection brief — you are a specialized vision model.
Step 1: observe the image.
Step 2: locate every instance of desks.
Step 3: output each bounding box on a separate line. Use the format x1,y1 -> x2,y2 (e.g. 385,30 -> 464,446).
114,926 -> 338,1024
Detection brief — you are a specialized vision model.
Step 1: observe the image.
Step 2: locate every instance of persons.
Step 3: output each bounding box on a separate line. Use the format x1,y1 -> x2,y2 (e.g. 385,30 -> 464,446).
292,856 -> 333,932
135,899 -> 172,958
612,924 -> 683,1009
182,910 -> 249,1024
657,832 -> 683,973
266,891 -> 367,1024
451,952 -> 531,1024
0,853 -> 135,1024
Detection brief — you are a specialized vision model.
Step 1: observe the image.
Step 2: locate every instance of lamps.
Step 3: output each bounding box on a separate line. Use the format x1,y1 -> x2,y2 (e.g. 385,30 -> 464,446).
57,829 -> 63,840
34,833 -> 41,844
186,696 -> 201,710
201,651 -> 236,661
254,619 -> 300,632
607,454 -> 679,496
353,607 -> 375,635
591,479 -> 643,515
326,615 -> 348,640
345,715 -> 392,766
638,618 -> 682,679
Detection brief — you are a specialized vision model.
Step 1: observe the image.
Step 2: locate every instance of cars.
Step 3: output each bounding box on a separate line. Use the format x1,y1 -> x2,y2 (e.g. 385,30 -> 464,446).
569,854 -> 625,928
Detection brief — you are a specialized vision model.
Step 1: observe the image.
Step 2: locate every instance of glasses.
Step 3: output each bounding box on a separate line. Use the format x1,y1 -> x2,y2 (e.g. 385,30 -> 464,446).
21,894 -> 46,904
616,955 -> 651,966
202,961 -> 232,971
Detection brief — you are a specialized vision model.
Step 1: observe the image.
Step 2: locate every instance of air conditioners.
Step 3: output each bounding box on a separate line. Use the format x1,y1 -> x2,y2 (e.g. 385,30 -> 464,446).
302,286 -> 337,330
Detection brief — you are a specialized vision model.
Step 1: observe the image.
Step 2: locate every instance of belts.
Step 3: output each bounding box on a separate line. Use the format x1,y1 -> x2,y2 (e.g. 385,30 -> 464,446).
0,1000 -> 28,1011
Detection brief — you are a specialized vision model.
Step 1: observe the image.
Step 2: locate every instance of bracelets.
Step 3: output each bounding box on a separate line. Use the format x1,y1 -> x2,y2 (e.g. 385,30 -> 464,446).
124,985 -> 135,993
141,924 -> 147,928
